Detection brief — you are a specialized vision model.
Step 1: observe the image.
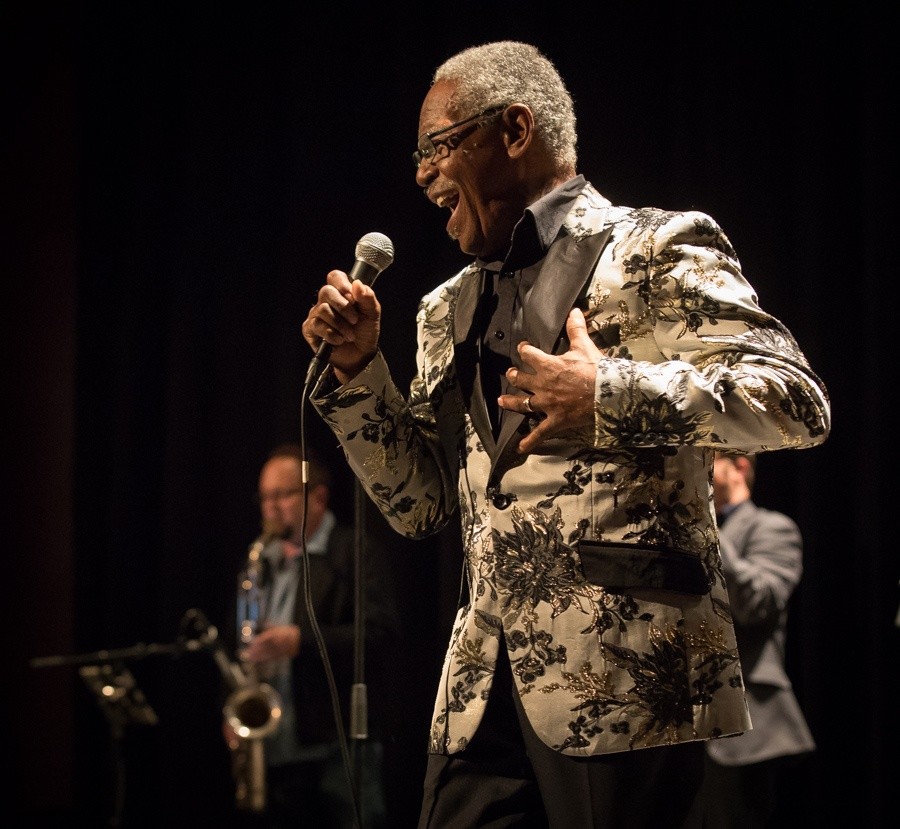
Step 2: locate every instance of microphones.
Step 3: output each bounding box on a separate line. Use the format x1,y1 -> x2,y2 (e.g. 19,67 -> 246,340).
304,231 -> 395,389
192,614 -> 230,677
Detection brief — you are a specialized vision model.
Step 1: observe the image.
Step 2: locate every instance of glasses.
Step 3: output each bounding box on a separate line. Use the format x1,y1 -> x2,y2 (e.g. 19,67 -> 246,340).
258,485 -> 309,501
411,102 -> 506,169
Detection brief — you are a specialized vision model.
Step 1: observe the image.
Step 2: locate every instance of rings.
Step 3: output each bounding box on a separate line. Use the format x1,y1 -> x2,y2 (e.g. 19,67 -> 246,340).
522,396 -> 533,413
507,370 -> 518,385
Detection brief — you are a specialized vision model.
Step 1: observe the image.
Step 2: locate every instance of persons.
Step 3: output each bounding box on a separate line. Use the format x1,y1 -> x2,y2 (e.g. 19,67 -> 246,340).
689,451 -> 818,829
299,41 -> 832,829
229,453 -> 397,829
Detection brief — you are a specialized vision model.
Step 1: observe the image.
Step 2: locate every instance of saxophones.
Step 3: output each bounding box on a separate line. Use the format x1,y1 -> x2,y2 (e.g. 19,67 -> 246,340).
221,530 -> 289,810
